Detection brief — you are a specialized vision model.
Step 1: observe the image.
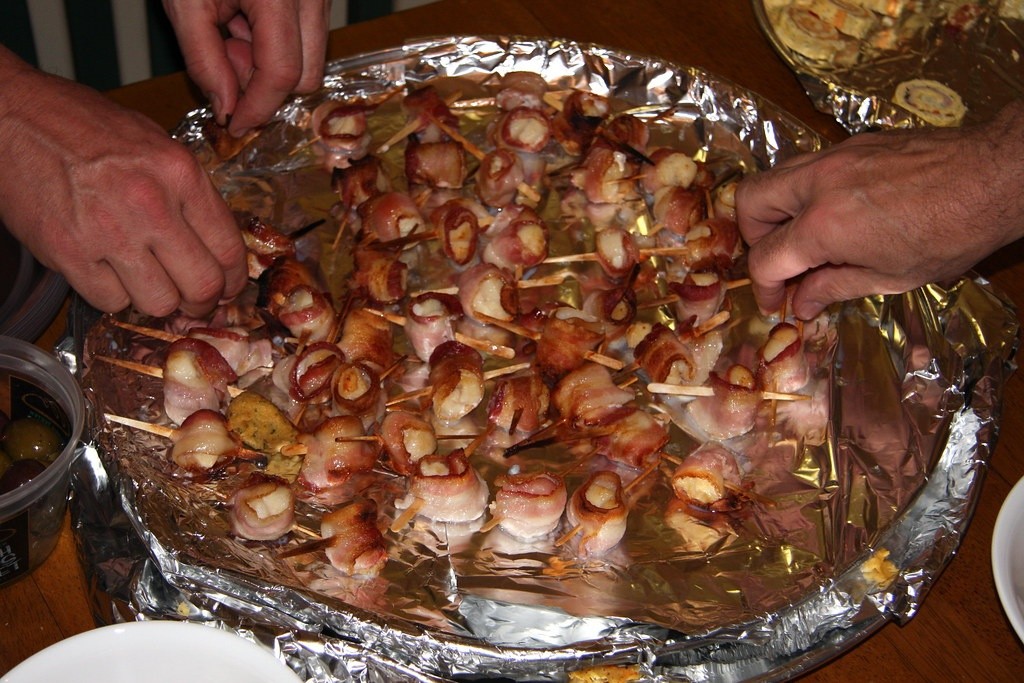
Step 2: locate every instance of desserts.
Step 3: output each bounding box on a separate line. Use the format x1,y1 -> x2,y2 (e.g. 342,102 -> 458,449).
770,0 -> 1024,130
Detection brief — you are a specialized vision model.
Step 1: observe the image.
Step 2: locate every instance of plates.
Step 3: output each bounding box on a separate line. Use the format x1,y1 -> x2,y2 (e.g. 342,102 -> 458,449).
0,620 -> 302,683
991,475 -> 1024,646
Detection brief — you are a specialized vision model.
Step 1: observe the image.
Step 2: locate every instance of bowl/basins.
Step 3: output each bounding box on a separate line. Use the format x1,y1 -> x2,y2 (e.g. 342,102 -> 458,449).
0,335 -> 85,587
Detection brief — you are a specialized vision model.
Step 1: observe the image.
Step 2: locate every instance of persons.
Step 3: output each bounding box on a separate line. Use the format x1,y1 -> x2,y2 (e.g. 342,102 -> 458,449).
0,0 -> 332,317
735,93 -> 1024,321
